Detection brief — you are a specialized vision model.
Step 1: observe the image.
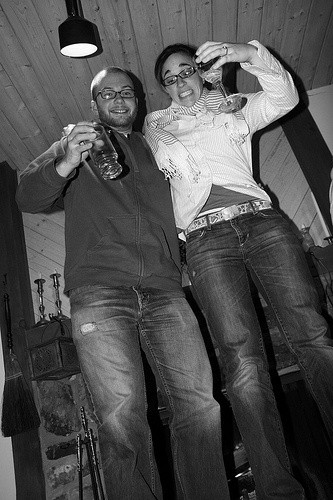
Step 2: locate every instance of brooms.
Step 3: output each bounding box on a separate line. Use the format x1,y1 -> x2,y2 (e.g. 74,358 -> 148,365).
1,292 -> 41,438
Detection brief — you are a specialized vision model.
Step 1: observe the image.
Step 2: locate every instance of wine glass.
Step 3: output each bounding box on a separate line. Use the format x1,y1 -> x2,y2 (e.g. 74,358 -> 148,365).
193,54 -> 242,113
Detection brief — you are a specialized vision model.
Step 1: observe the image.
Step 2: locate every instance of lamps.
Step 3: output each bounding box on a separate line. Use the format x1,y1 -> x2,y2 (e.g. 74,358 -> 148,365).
58,0 -> 99,59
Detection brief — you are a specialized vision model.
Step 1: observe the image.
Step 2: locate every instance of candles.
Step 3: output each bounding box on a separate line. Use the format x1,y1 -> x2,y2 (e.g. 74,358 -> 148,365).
39,273 -> 42,279
53,268 -> 57,274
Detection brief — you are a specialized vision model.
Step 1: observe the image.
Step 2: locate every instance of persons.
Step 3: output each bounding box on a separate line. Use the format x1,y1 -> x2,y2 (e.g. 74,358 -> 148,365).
15,66 -> 264,500
62,40 -> 333,500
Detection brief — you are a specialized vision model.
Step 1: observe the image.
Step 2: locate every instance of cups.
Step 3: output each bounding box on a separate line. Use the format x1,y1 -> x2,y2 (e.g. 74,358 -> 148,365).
81,124 -> 123,180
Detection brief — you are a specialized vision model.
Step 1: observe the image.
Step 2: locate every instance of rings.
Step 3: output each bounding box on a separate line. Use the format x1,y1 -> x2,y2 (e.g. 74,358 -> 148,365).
223,46 -> 228,55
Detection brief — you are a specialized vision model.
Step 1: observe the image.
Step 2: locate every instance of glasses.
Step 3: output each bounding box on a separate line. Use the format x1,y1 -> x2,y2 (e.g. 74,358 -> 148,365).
95,89 -> 136,98
163,66 -> 198,87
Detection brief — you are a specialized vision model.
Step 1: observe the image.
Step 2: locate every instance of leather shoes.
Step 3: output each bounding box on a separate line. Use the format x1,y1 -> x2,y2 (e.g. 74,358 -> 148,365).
186,200 -> 272,233
